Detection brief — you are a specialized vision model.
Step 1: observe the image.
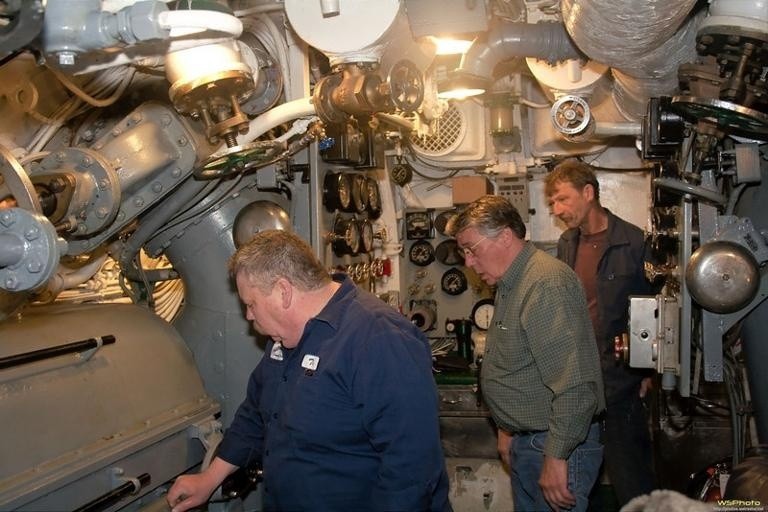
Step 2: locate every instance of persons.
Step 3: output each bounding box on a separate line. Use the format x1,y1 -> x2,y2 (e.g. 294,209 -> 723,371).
543,154 -> 668,504
164,228 -> 454,511
722,456 -> 768,511
451,193 -> 610,512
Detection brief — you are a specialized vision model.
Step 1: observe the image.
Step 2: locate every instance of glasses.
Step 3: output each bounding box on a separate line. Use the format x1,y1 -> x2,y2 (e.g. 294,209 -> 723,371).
457,237 -> 487,258
